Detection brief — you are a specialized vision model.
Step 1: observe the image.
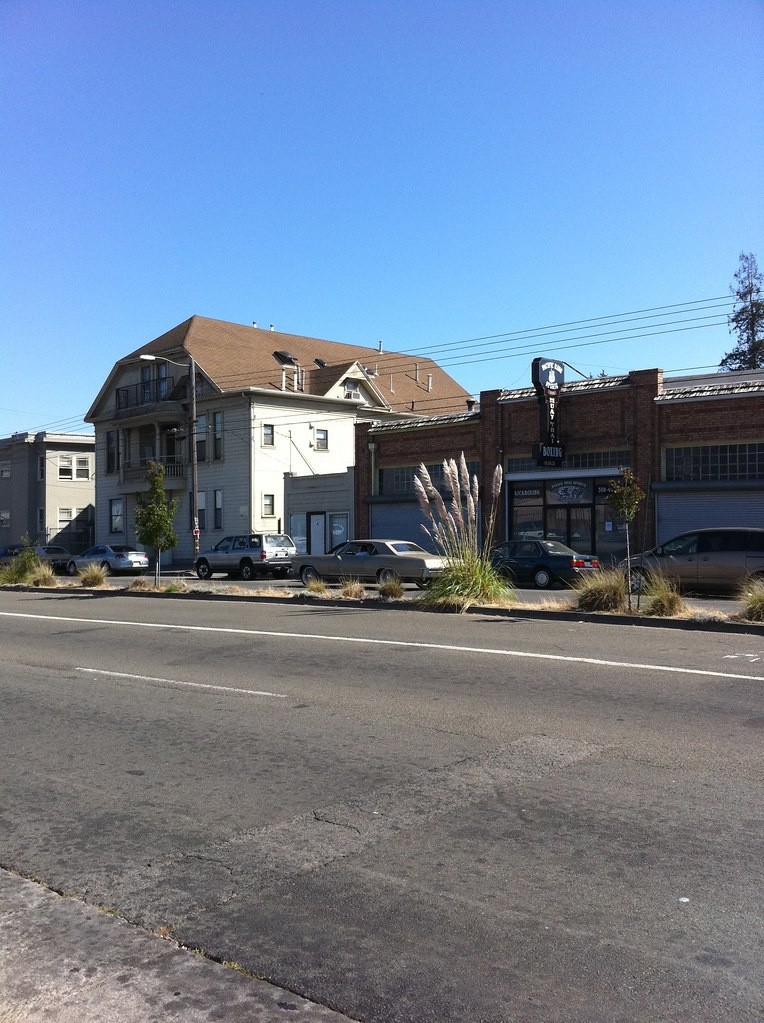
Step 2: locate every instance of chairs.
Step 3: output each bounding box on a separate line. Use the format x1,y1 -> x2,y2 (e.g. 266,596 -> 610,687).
235,541 -> 246,550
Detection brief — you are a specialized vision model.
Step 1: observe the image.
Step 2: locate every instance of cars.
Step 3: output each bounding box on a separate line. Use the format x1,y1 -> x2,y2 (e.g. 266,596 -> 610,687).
481,540 -> 601,587
567,530 -> 628,556
1,546 -> 80,571
291,539 -> 465,589
66,544 -> 149,576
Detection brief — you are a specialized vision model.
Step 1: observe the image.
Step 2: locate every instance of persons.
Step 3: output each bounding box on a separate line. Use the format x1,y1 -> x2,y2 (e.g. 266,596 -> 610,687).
355,545 -> 370,556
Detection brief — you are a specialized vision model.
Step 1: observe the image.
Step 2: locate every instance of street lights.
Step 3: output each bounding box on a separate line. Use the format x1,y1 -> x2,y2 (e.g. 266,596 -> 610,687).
140,354 -> 201,560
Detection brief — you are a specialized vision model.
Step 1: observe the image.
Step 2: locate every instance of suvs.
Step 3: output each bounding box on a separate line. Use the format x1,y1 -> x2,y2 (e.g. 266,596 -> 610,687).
195,533 -> 299,580
292,537 -> 307,552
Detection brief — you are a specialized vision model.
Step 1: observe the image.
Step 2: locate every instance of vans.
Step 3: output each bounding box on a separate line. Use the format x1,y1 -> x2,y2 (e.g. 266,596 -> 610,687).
616,527 -> 764,595
